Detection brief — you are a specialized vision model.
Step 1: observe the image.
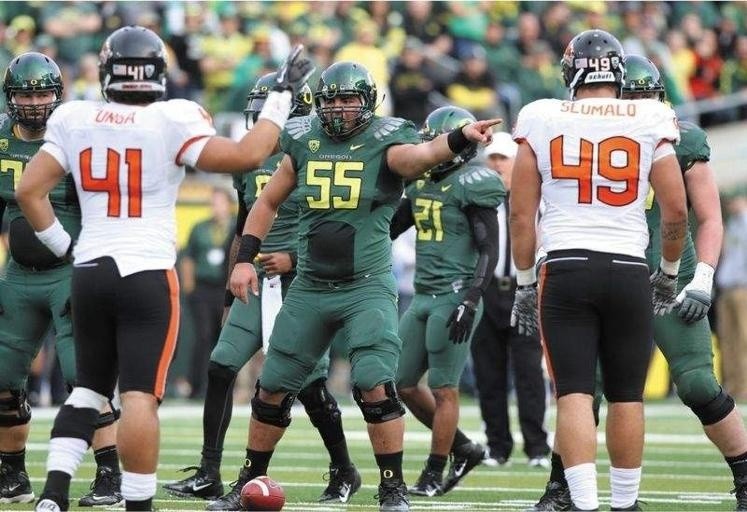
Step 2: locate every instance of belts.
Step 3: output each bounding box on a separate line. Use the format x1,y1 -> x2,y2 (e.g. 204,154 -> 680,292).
489,277 -> 516,293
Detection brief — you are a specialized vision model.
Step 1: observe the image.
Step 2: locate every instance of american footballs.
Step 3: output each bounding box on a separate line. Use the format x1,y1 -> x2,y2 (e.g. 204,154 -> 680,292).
240,478 -> 285,509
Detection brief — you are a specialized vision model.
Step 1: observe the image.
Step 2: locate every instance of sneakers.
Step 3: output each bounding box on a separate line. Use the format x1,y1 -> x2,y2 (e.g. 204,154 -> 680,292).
480,455 -> 745,511
205,474 -> 252,510
162,464 -> 223,500
406,467 -> 445,497
441,440 -> 490,491
0,462 -> 34,506
317,463 -> 361,503
77,466 -> 121,507
373,478 -> 408,512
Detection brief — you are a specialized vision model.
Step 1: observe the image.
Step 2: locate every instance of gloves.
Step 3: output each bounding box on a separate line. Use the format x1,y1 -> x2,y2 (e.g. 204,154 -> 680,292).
649,265 -> 678,316
675,273 -> 714,323
445,302 -> 476,343
509,281 -> 539,337
270,44 -> 316,108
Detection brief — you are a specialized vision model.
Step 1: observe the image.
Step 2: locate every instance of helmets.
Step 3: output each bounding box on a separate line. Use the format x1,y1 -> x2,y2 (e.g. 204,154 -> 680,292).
314,62 -> 378,141
244,74 -> 312,130
418,106 -> 479,183
2,52 -> 63,134
560,30 -> 627,101
621,54 -> 667,103
97,25 -> 168,102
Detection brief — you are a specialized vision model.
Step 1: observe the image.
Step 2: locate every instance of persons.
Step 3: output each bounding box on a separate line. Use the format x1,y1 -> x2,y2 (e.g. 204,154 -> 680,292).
201,60 -> 503,511
508,30 -> 687,511
0,53 -> 121,506
162,70 -> 362,503
0,1 -> 747,405
389,104 -> 508,497
14,25 -> 317,511
525,53 -> 747,512
468,130 -> 554,469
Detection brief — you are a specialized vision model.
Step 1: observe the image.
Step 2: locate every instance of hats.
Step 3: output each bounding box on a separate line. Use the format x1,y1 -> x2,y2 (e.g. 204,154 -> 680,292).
484,132 -> 519,158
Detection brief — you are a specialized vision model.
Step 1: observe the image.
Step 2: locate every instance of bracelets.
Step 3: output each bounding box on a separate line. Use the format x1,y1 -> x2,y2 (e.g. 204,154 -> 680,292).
446,124 -> 471,153
260,89 -> 292,130
658,255 -> 681,279
514,266 -> 537,286
34,221 -> 73,259
224,290 -> 235,307
235,234 -> 261,264
